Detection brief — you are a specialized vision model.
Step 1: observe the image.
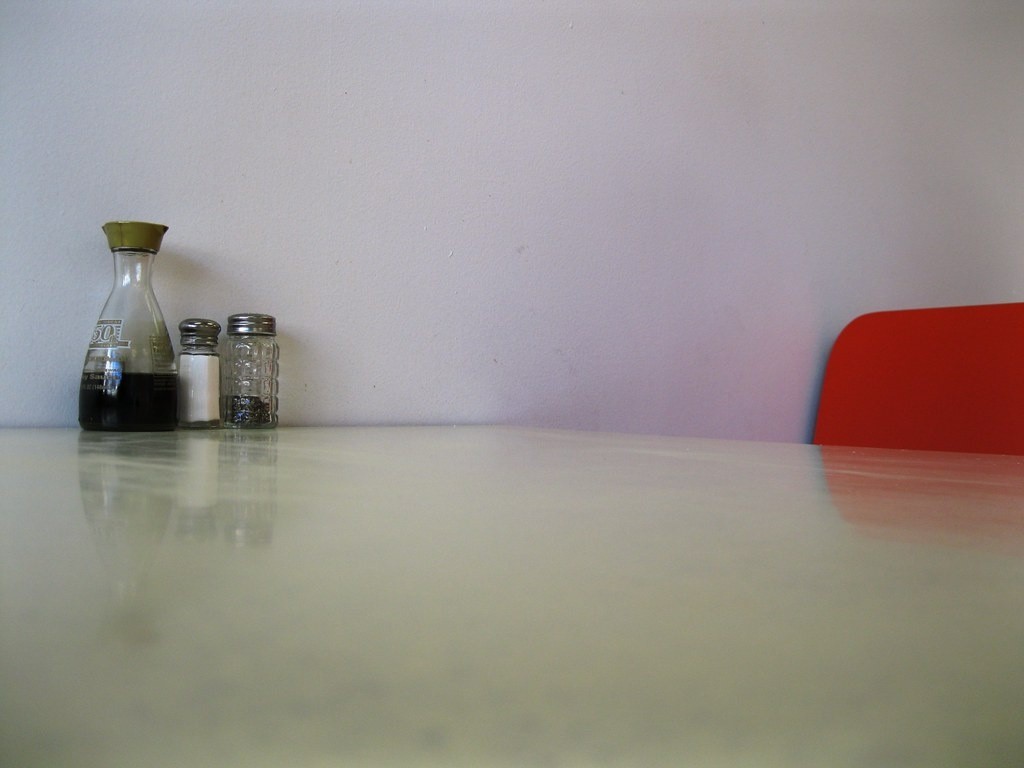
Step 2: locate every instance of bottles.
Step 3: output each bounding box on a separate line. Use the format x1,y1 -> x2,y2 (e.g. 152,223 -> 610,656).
222,314 -> 280,428
178,318 -> 221,427
78,221 -> 178,430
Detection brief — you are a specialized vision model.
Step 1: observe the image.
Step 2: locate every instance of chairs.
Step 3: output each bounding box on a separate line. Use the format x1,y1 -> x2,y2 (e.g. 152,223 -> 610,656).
810,301 -> 1024,456
